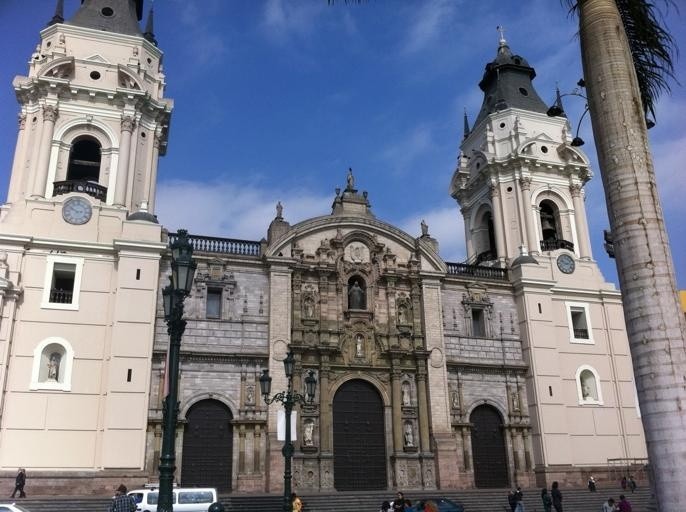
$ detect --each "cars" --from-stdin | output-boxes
[0,503,31,512]
[411,497,464,512]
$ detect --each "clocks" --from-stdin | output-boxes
[556,254,575,274]
[61,199,92,226]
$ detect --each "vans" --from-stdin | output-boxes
[126,488,218,512]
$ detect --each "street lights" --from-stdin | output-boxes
[259,352,317,512]
[547,78,655,146]
[157,229,198,512]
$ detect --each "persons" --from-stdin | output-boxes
[304,423,314,445]
[133,493,141,503]
[290,492,302,512]
[405,424,413,447]
[621,477,627,489]
[348,281,365,308]
[514,486,525,511]
[542,487,552,512]
[19,469,26,497]
[552,481,563,512]
[304,300,315,317]
[398,306,408,323]
[48,354,57,379]
[10,468,24,497]
[380,491,440,511]
[588,476,596,493]
[208,502,225,511]
[421,219,428,234]
[613,494,632,512]
[628,476,636,493]
[403,387,411,406]
[347,167,355,189]
[601,499,616,511]
[276,199,283,217]
[108,483,138,512]
[355,335,364,355]
[508,490,516,511]
